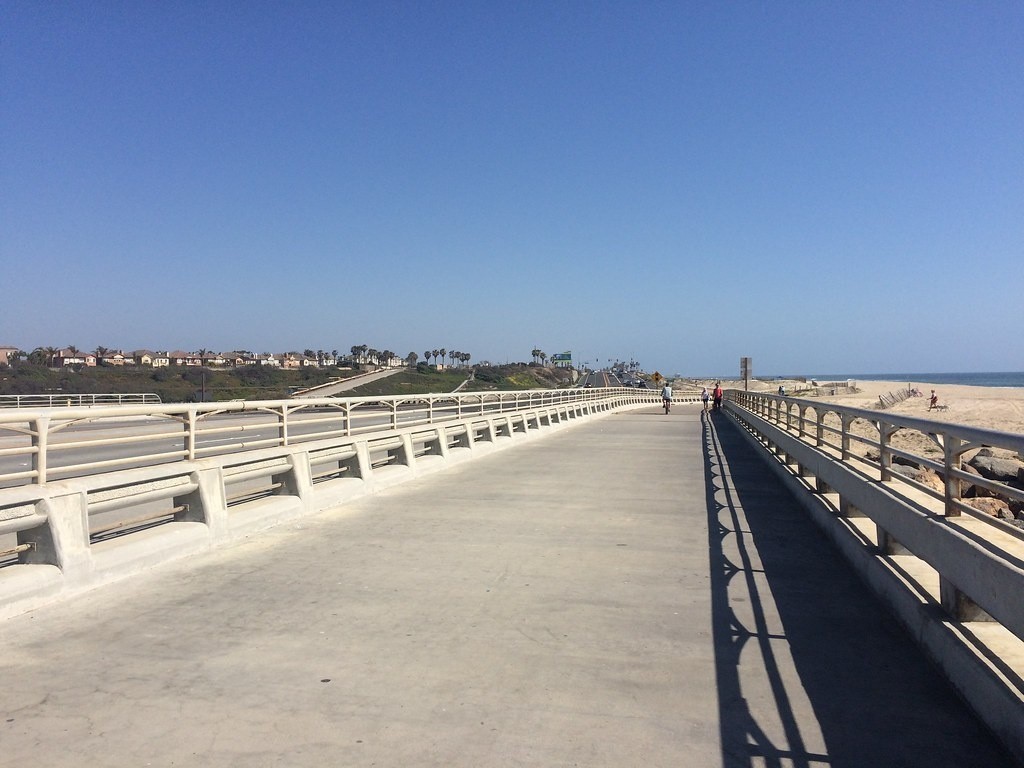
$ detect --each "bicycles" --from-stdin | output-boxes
[661,394,673,415]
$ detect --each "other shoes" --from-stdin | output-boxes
[663,405,665,408]
[668,408,670,412]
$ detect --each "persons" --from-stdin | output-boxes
[660,382,672,412]
[701,387,709,409]
[779,386,785,395]
[712,383,722,414]
[927,389,939,412]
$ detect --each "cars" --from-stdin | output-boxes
[590,369,646,389]
[585,382,593,388]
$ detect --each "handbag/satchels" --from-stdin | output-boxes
[933,396,938,403]
